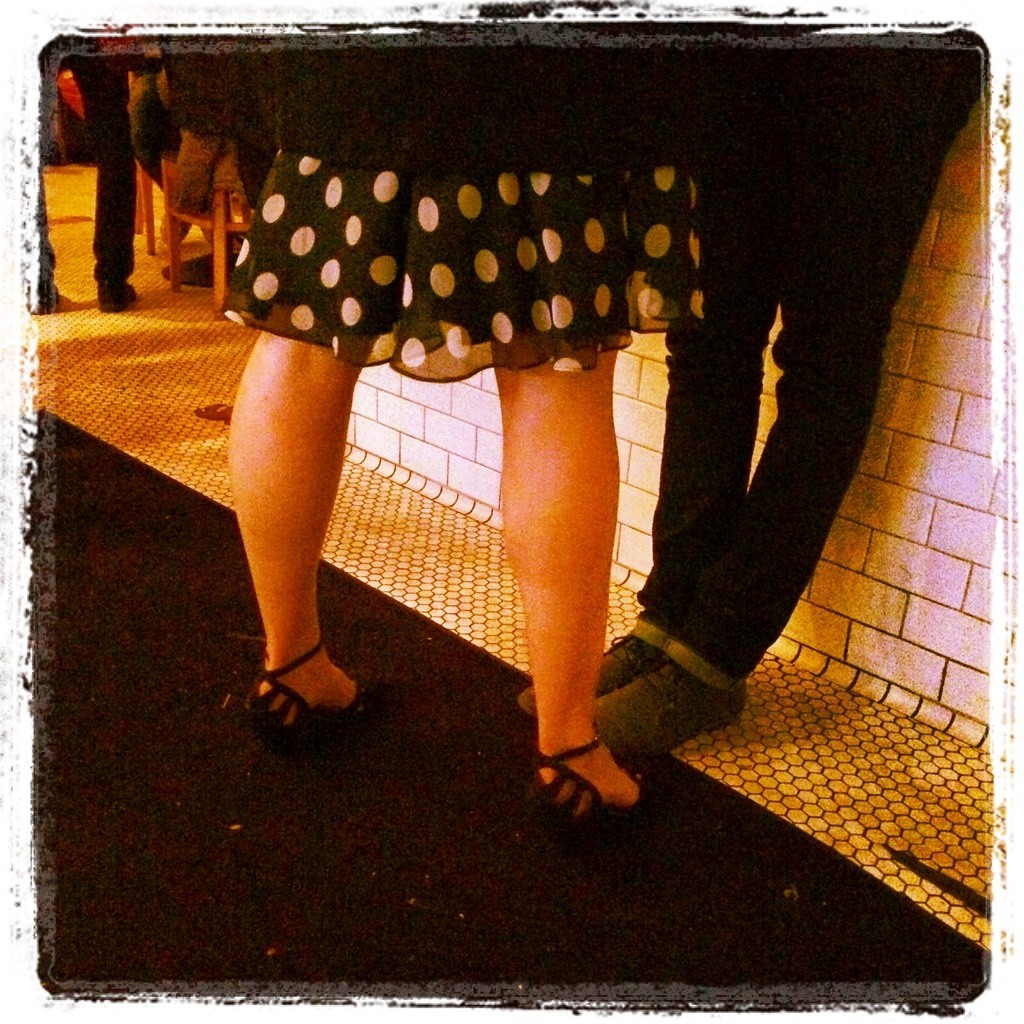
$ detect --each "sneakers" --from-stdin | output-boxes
[593,655,744,750]
[516,635,673,719]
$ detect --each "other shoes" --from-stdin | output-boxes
[97,279,136,313]
[39,280,58,313]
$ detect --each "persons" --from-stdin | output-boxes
[226,50,700,835]
[35,51,278,316]
[515,49,981,758]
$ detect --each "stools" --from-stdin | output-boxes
[162,147,266,315]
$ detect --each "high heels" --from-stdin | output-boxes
[534,737,674,859]
[250,638,385,751]
